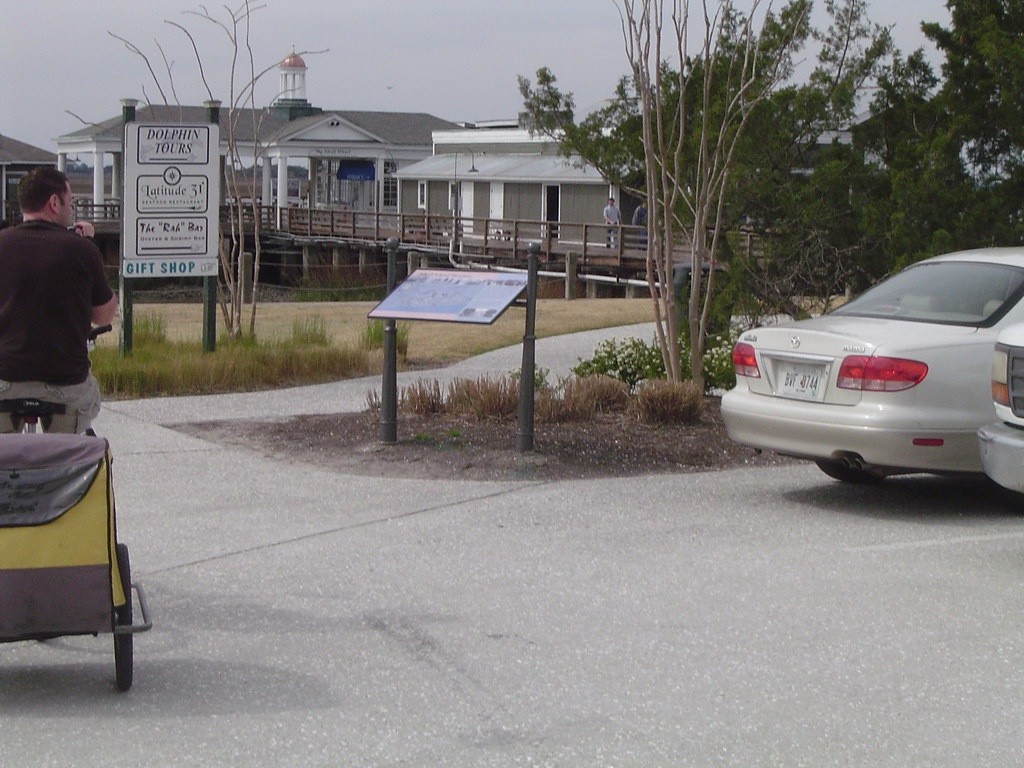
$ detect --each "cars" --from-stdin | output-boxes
[719,245,1024,486]
[979,323,1024,496]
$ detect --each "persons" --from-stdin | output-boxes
[604,198,623,248]
[632,199,648,252]
[0,167,116,434]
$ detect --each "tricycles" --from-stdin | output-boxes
[0,321,153,692]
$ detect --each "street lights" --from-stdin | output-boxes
[374,150,396,243]
[454,148,478,253]
